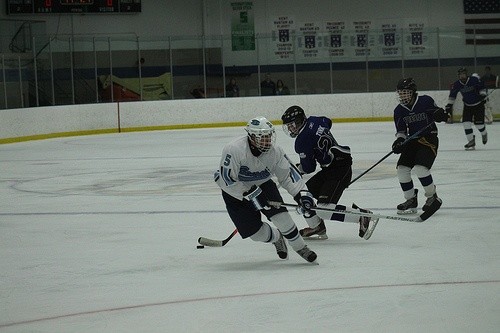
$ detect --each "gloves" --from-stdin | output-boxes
[242,184,271,210]
[434,109,450,122]
[299,190,313,217]
[392,138,405,154]
[444,104,453,115]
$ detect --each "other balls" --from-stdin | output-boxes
[197,246,204,249]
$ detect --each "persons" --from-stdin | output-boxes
[392,77,445,215]
[261,73,290,95]
[446,68,492,150]
[214,115,318,265]
[482,67,496,88]
[226,79,240,96]
[281,105,379,240]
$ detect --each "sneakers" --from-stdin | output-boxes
[351,203,378,240]
[463,135,475,150]
[296,247,319,266]
[396,189,419,215]
[422,193,437,211]
[482,134,487,145]
[299,219,330,240]
[274,229,288,260]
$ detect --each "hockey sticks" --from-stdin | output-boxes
[198,184,282,247]
[344,120,436,189]
[483,88,496,100]
[268,197,442,223]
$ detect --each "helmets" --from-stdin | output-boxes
[458,67,467,81]
[244,116,275,153]
[281,106,305,139]
[396,78,416,106]
